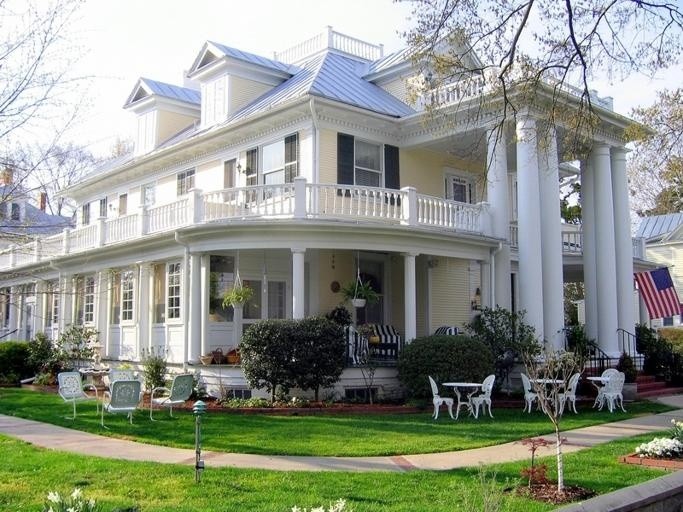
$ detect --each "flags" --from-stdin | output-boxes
[632,266,682,321]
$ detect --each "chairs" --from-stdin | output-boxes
[58,369,193,430]
[427,368,628,421]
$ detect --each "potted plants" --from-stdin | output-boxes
[222,286,254,310]
[140,345,168,406]
[341,277,379,307]
